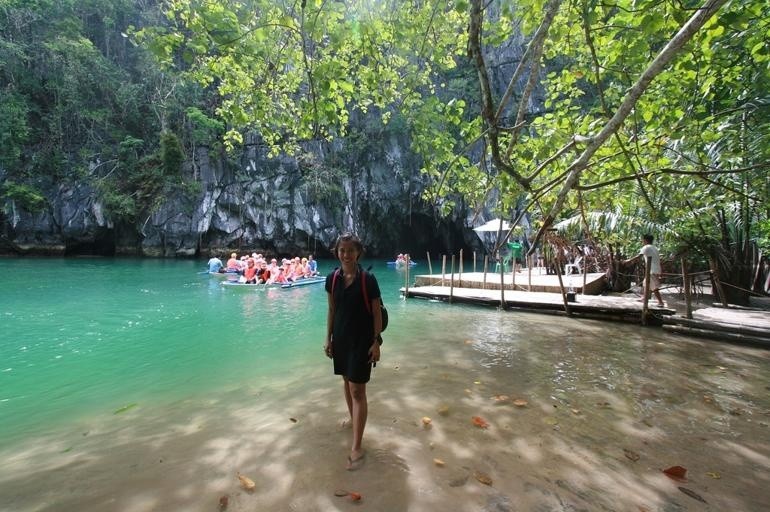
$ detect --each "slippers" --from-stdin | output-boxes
[348,455,364,471]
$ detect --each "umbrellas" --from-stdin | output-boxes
[470,216,522,259]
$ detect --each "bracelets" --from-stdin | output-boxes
[373,336,379,341]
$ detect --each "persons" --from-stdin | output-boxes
[621,233,665,308]
[396,264,411,274]
[535,246,548,272]
[395,251,405,263]
[403,253,415,263]
[245,289,310,318]
[323,230,383,471]
[208,251,320,286]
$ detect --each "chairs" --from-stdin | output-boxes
[495,255,510,273]
[565,255,582,277]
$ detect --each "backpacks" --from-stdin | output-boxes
[331,269,387,332]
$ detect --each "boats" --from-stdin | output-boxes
[220,276,328,288]
[386,260,417,266]
[209,266,237,275]
